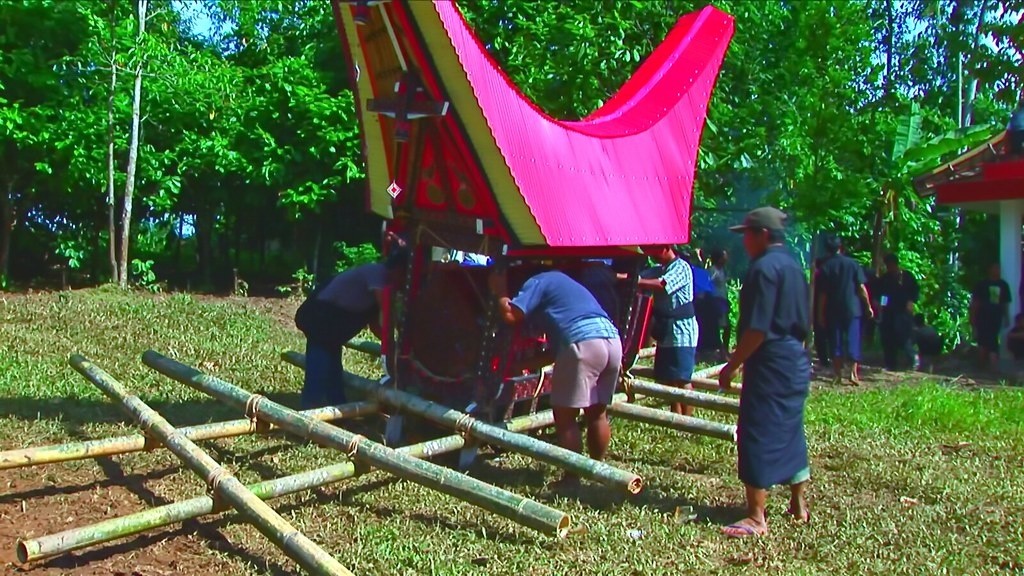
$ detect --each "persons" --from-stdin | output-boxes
[810,233,944,387]
[969,261,1024,375]
[719,207,810,538]
[488,265,624,493]
[637,245,732,418]
[295,247,408,429]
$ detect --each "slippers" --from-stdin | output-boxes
[781,508,810,524]
[724,515,768,537]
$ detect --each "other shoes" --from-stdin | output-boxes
[850,373,859,387]
[547,477,581,488]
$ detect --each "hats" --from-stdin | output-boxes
[827,237,842,247]
[731,207,786,230]
